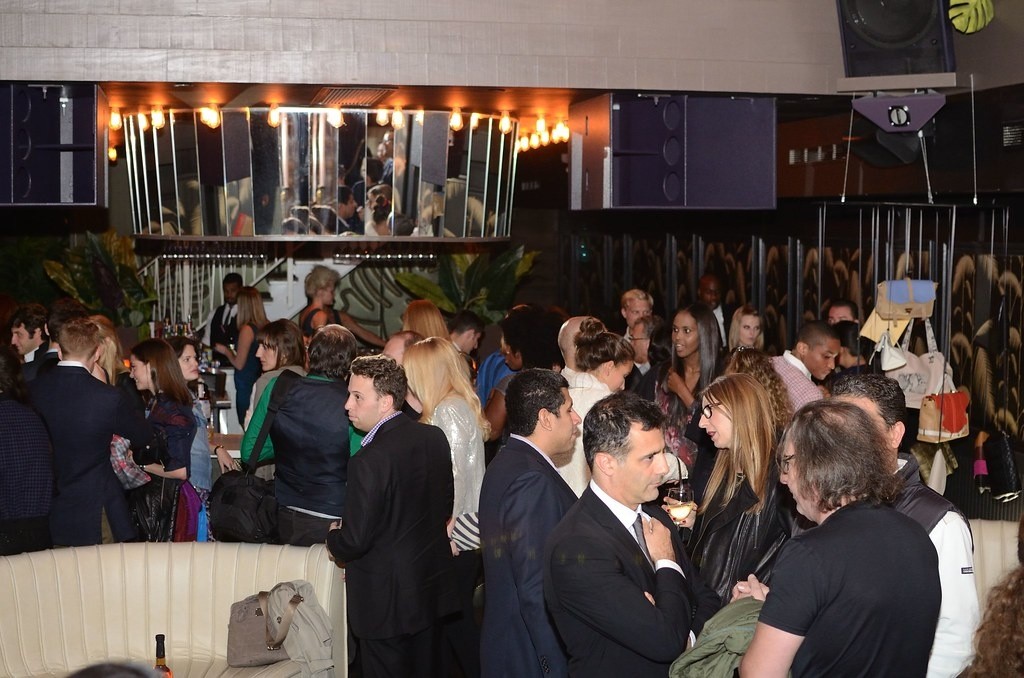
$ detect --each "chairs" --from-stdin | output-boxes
[910,441,980,514]
[969,517,1020,618]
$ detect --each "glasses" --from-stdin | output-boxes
[628,334,647,342]
[702,401,724,418]
[781,448,799,474]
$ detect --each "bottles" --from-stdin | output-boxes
[164,315,196,338]
[153,634,173,677]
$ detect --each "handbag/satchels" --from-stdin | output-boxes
[226,591,296,667]
[876,280,937,320]
[884,318,954,409]
[209,470,278,543]
[875,334,907,372]
[917,373,969,444]
[910,440,959,485]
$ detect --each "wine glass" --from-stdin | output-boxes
[668,488,694,530]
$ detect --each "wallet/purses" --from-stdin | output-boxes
[983,433,1022,502]
[974,431,990,494]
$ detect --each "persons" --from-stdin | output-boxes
[0,114,1024,678]
[328,356,458,678]
[478,368,582,677]
[544,391,722,677]
[728,399,943,678]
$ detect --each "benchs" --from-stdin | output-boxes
[0,540,350,678]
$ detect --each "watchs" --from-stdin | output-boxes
[214,444,225,455]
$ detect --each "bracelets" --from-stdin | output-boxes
[139,464,145,471]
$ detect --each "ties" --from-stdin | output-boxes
[632,513,652,567]
[224,305,233,325]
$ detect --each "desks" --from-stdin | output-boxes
[207,434,245,488]
[144,391,231,434]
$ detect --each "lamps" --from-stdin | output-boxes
[108,106,572,163]
